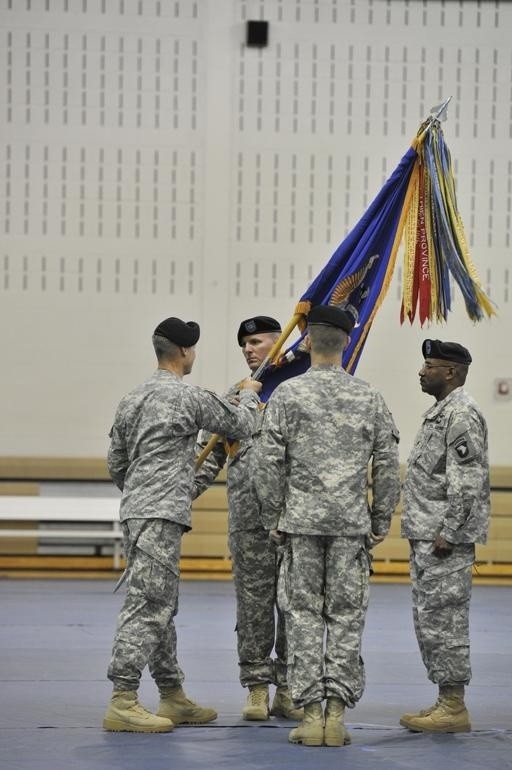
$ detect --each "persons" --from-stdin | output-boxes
[400,338,492,736]
[256,307,399,749]
[188,311,311,726]
[97,315,263,737]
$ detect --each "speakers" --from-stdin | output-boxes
[247,20,269,45]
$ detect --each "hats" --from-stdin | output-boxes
[307,305,352,335]
[154,317,200,347]
[238,316,282,347]
[422,339,472,365]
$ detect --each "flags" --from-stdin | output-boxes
[294,140,422,377]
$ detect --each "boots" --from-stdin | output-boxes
[243,683,271,721]
[288,702,325,746]
[399,686,445,728]
[155,685,218,725]
[407,684,471,734]
[102,689,174,733]
[270,686,305,721]
[323,697,351,747]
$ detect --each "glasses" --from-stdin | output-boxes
[422,364,452,369]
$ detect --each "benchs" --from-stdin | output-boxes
[1,492,128,572]
